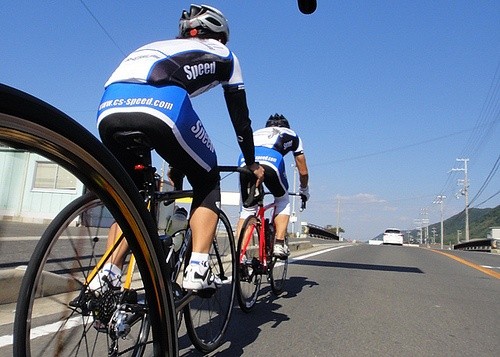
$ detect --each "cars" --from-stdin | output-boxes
[382,228,404,246]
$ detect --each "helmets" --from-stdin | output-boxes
[266,113,290,129]
[180,4,229,43]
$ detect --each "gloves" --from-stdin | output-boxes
[298,187,309,201]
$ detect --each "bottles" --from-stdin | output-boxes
[264,218,274,252]
[159,234,176,273]
[165,207,188,252]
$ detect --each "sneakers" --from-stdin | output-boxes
[273,245,290,256]
[183,261,223,291]
[239,255,249,276]
[87,270,122,294]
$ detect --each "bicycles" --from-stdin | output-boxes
[0,81,311,357]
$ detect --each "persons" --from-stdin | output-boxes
[237,113,309,278]
[84,4,265,329]
